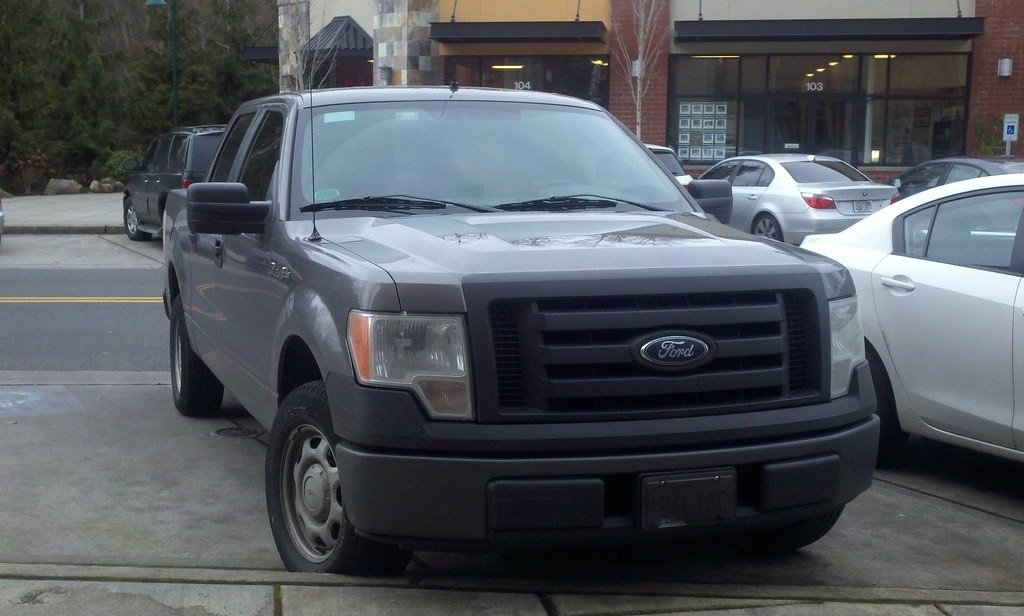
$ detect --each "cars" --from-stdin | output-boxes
[889,157,1024,236]
[801,175,1024,466]
[696,152,899,247]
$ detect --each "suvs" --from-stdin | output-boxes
[643,144,694,189]
[162,87,880,575]
[122,124,226,241]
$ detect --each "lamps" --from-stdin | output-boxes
[632,59,646,78]
[378,64,392,79]
[280,72,292,88]
[997,57,1013,77]
